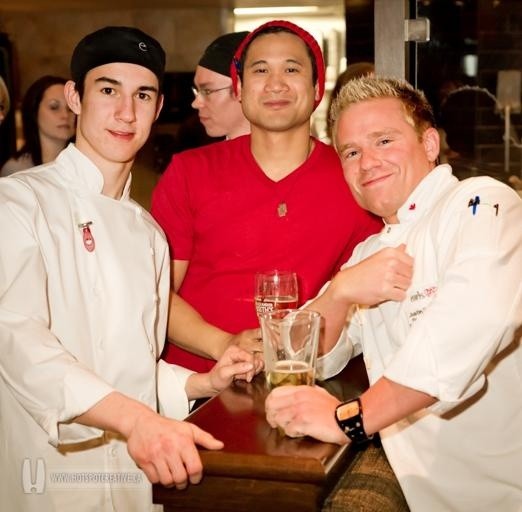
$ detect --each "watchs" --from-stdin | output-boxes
[333,397,376,445]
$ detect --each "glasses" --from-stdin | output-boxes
[192,84,233,100]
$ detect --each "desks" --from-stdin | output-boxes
[153,375,370,511]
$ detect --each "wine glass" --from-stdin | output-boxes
[253,269,299,360]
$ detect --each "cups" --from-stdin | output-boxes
[254,309,323,439]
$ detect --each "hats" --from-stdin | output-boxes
[198,31,251,78]
[71,26,165,90]
[231,20,325,113]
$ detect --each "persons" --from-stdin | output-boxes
[0,73,77,178]
[191,30,254,141]
[149,19,385,408]
[326,61,374,139]
[265,73,522,511]
[440,84,522,197]
[1,23,270,512]
